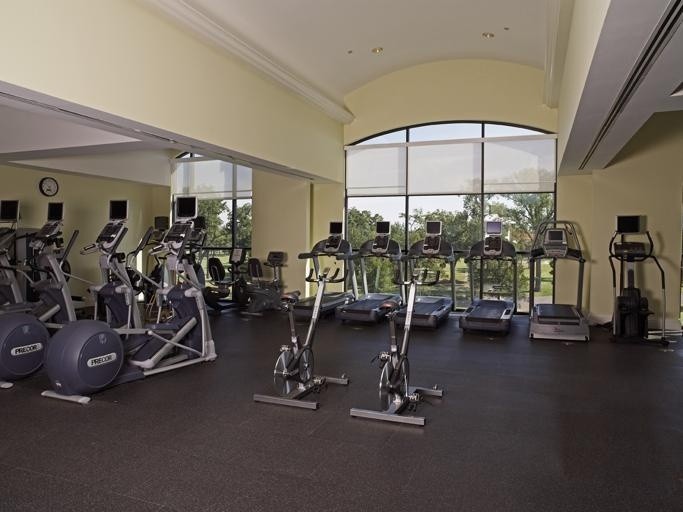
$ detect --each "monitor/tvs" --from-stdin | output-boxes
[155,216,169,229]
[485,220,502,235]
[615,214,643,235]
[228,247,246,265]
[109,199,129,221]
[543,229,568,245]
[375,221,391,236]
[47,201,65,221]
[328,220,344,235]
[190,215,206,229]
[0,200,20,222]
[425,220,442,236]
[174,194,198,219]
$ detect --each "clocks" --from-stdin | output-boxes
[39,177,59,196]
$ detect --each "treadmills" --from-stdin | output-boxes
[289,222,352,320]
[389,221,455,328]
[529,221,590,344]
[459,221,517,336]
[336,221,402,327]
